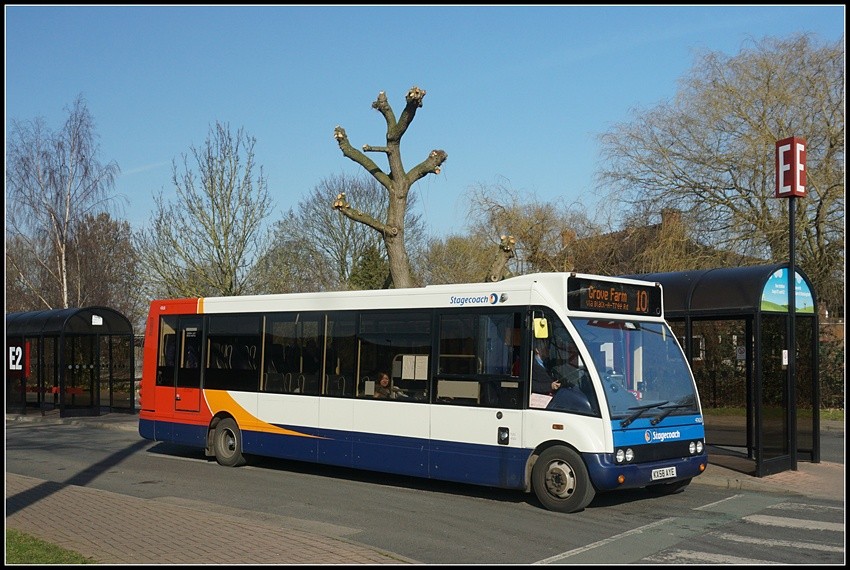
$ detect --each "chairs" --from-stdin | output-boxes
[164,334,372,398]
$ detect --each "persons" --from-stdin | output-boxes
[531,341,564,396]
[372,372,395,397]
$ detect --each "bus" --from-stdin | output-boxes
[138,271,709,512]
